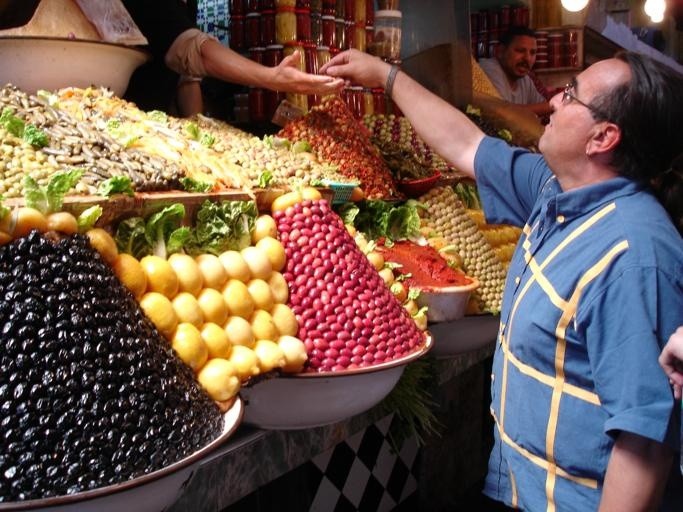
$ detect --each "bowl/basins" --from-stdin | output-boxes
[241,331,436,430]
[1,35,149,100]
[426,312,501,360]
[393,167,443,198]
[415,276,480,323]
[1,391,245,511]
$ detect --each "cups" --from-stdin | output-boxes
[547,33,563,66]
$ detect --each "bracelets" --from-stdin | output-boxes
[385,57,404,99]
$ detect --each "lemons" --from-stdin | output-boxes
[466,209,523,273]
[0,187,322,413]
[344,225,428,332]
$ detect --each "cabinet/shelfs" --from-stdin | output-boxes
[476,20,583,73]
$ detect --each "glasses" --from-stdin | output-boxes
[562,83,612,121]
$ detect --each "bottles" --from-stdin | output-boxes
[469,5,511,59]
[227,1,402,139]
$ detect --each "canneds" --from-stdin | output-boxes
[228,0,404,123]
[471,4,528,59]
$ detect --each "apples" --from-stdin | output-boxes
[498,129,512,142]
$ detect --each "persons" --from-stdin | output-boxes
[316,48,683,511]
[122,1,346,130]
[476,26,554,124]
[658,323,683,400]
